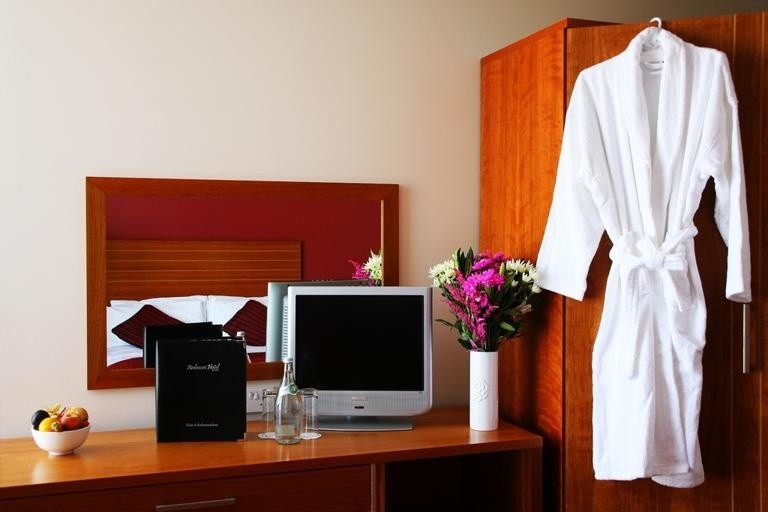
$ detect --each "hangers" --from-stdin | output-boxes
[634,16,675,67]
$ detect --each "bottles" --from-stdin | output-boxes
[275,358,301,444]
[237,330,251,363]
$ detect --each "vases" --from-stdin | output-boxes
[467,347,501,433]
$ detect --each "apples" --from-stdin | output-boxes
[62,413,79,430]
[72,407,88,427]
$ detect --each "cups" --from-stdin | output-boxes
[263,389,276,437]
[301,389,319,439]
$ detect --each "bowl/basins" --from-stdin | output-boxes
[31,421,92,456]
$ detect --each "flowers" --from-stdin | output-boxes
[347,248,383,287]
[427,247,542,354]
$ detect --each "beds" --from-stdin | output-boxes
[96,237,302,388]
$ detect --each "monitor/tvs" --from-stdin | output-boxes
[265,279,382,364]
[287,286,434,431]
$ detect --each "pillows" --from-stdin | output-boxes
[110,297,203,327]
[111,304,184,349]
[206,295,268,331]
[222,299,267,347]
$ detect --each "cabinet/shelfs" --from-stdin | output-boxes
[0,404,544,511]
[481,12,767,511]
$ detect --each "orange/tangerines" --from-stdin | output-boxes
[38,418,61,432]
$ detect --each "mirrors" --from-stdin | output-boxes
[84,176,399,391]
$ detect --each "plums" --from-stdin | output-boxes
[31,410,49,430]
